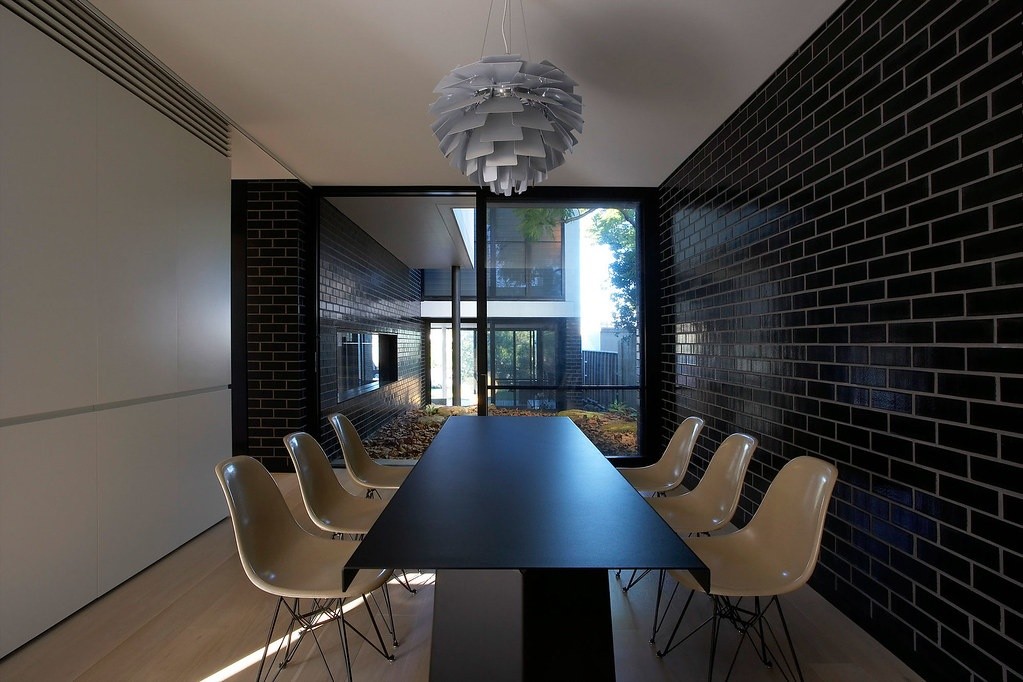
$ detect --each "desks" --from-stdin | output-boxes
[341,415,711,682]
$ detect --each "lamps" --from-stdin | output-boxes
[428,1,585,197]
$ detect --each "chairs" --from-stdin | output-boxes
[215,412,414,682]
[615,417,838,682]
